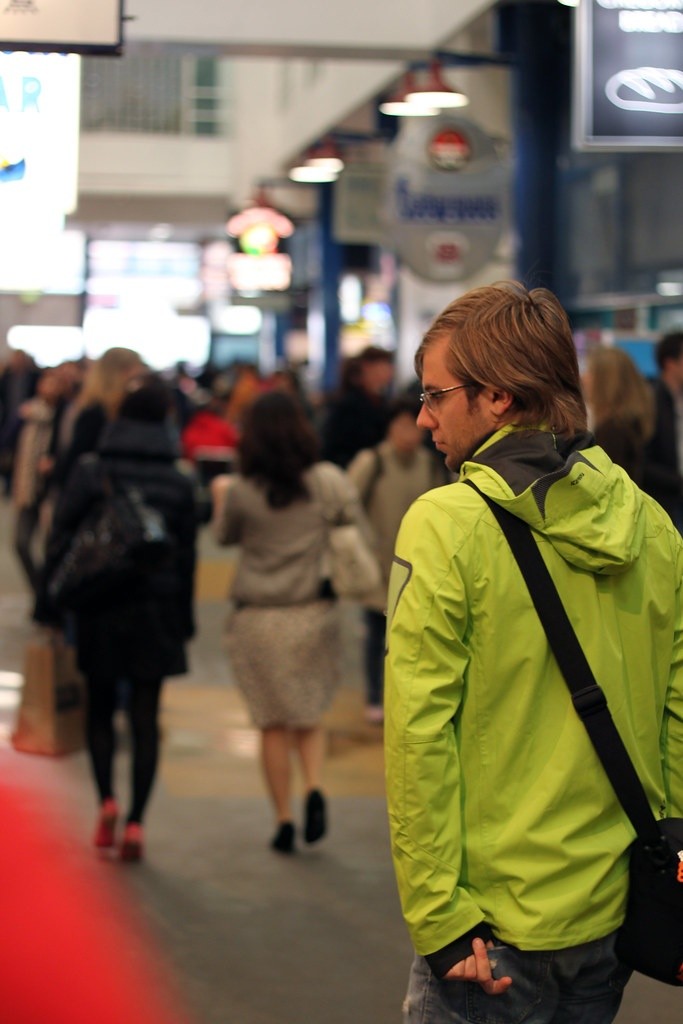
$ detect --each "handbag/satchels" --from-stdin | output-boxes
[615,817,683,986]
[13,626,81,756]
[320,464,378,600]
[43,473,151,605]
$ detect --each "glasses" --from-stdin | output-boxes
[420,384,482,414]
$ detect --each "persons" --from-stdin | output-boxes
[346,398,434,723]
[212,389,376,853]
[576,327,683,536]
[176,346,399,523]
[383,280,683,1024]
[0,347,148,502]
[30,386,200,863]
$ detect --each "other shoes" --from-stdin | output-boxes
[304,791,325,845]
[272,822,293,851]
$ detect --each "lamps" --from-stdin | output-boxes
[290,56,471,183]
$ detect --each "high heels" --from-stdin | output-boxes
[121,823,144,862]
[95,799,118,848]
